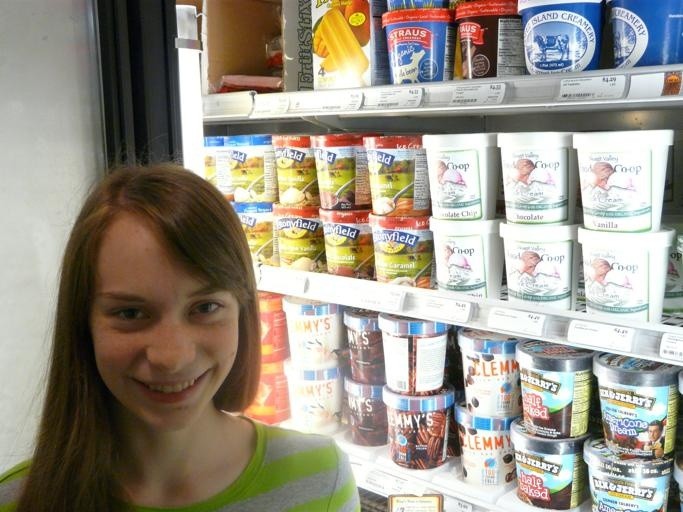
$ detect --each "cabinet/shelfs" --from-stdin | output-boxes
[198,0,683,512]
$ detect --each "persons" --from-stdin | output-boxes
[0,160,361,512]
[641,420,665,459]
